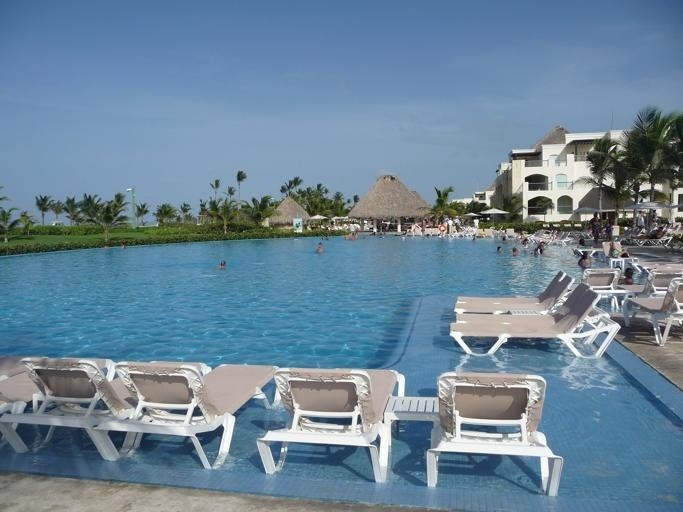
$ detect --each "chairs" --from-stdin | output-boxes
[448,223,682,358]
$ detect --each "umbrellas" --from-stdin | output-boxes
[306,214,328,225]
[622,201,681,225]
[479,207,511,226]
[575,205,597,221]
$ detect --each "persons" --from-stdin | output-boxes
[424,209,682,284]
[218,260,226,269]
[302,220,398,240]
[119,239,126,249]
[315,242,324,253]
